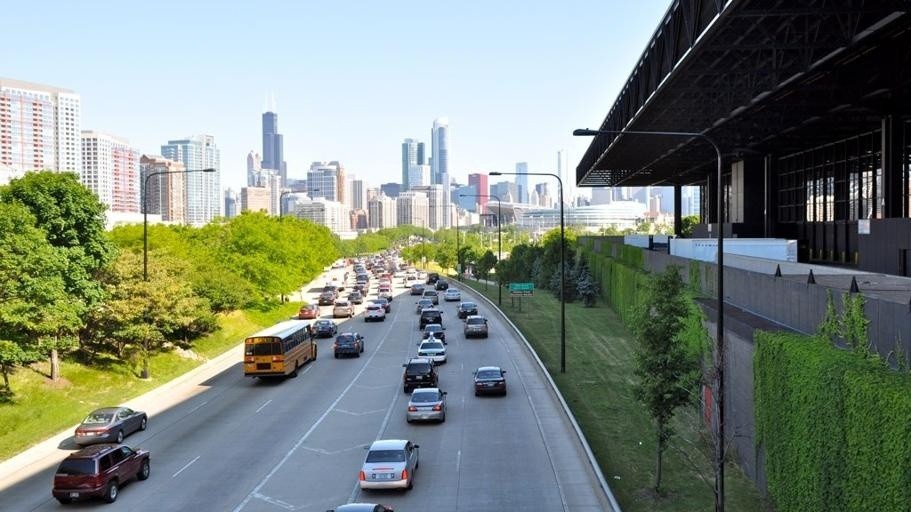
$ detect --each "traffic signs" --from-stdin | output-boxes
[509,282,535,297]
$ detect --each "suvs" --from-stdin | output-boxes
[333,331,365,360]
[418,307,445,329]
[402,356,443,392]
[49,442,153,505]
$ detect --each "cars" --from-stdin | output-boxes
[416,331,450,364]
[326,500,396,511]
[471,365,509,398]
[462,314,490,339]
[312,318,339,338]
[454,300,481,320]
[72,405,150,446]
[318,241,462,323]
[357,437,421,491]
[299,304,321,319]
[419,322,449,343]
[405,386,449,424]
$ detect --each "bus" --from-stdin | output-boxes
[240,317,319,381]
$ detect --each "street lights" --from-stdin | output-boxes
[488,171,568,374]
[297,207,327,219]
[460,193,504,306]
[279,187,322,302]
[140,166,220,381]
[571,126,732,511]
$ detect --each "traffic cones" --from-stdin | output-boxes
[774,263,783,276]
[849,275,861,293]
[808,268,818,283]
